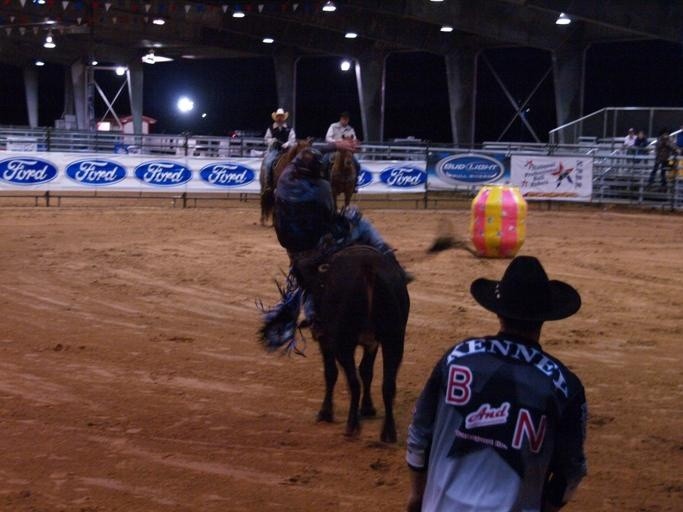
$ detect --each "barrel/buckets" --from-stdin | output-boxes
[466,184,530,257]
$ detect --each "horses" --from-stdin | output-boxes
[328,134,357,212]
[259,137,315,227]
[302,240,412,444]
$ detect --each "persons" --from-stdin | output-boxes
[623,128,637,147]
[647,129,682,194]
[325,203,384,253]
[275,139,356,253]
[326,111,358,144]
[627,130,649,162]
[263,108,296,183]
[409,255,587,512]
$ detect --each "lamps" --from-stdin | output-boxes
[322,0,336,11]
[43,29,57,49]
[555,12,571,24]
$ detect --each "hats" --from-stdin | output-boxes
[272,108,289,121]
[470,256,581,320]
